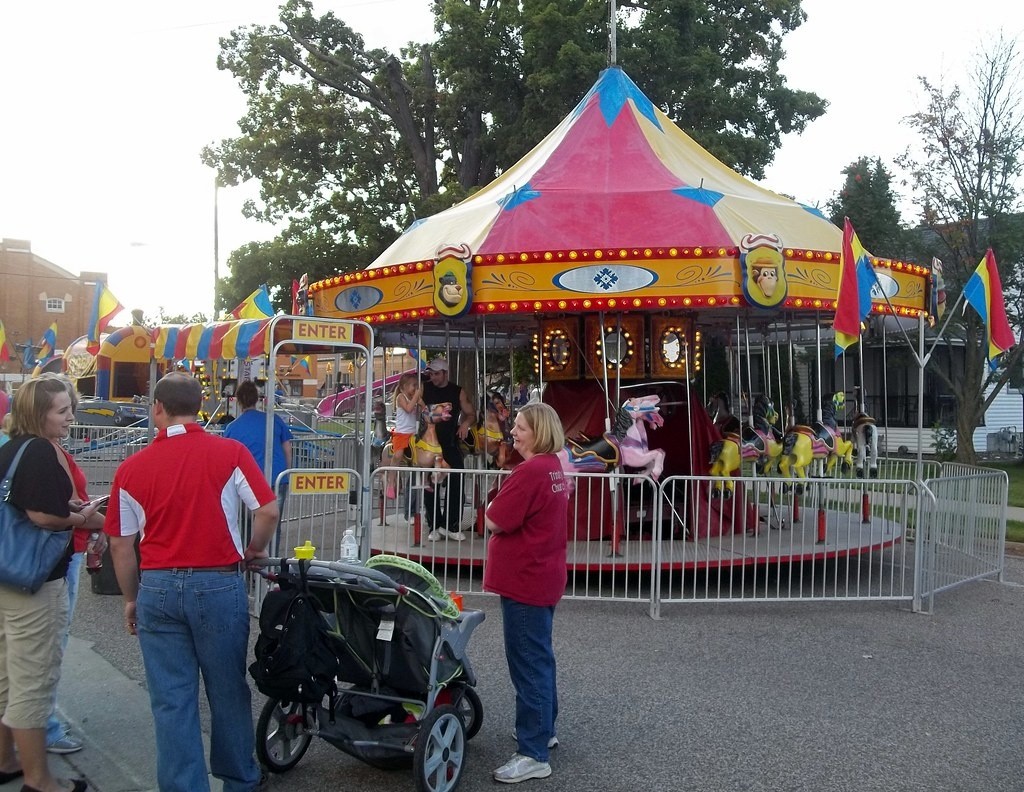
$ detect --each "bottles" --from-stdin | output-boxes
[87,533,102,575]
[340,529,358,564]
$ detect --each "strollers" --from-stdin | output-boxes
[246,542,486,792]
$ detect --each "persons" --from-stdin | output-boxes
[223,379,294,590]
[102,372,281,792]
[482,403,569,783]
[0,370,107,792]
[383,357,476,542]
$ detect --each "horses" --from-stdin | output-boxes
[455,388,513,468]
[373,402,451,497]
[554,394,666,495]
[706,389,880,501]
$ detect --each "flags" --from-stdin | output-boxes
[36,319,58,367]
[962,246,1015,372]
[232,283,275,322]
[290,354,298,365]
[22,337,35,370]
[85,276,125,356]
[0,319,10,363]
[830,215,877,362]
[409,348,427,370]
[300,354,313,376]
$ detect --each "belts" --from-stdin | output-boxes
[168,560,238,572]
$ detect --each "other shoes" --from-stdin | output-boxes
[20,778,87,792]
[386,486,395,499]
[47,735,83,753]
[251,769,275,792]
[55,720,72,733]
[0,770,23,785]
[425,486,434,493]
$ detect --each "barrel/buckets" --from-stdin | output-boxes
[90,500,142,595]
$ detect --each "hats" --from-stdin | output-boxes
[426,358,449,372]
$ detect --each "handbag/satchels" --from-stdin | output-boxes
[0,437,73,595]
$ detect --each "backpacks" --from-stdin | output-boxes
[249,558,341,727]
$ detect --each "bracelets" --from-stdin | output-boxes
[80,513,87,528]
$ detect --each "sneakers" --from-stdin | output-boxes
[428,529,441,541]
[439,526,466,541]
[493,752,551,784]
[512,728,559,749]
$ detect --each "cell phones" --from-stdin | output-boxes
[82,494,110,507]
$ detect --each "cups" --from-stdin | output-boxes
[293,541,316,559]
[450,592,463,611]
